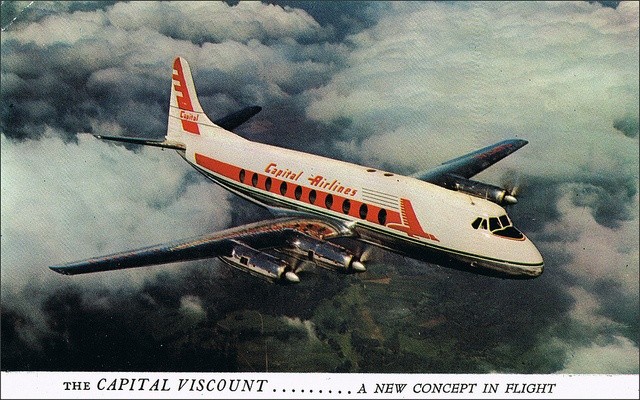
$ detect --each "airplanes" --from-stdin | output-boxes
[48,55,545,285]
